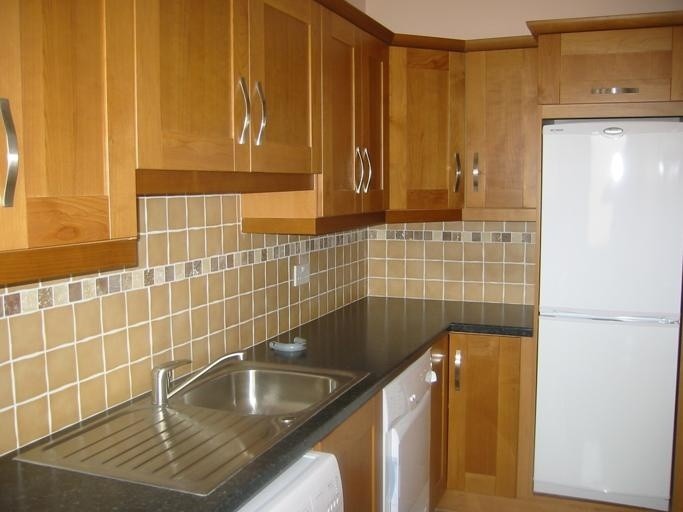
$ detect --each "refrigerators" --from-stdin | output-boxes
[532,117,683,512]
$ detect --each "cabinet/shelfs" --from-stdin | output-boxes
[318,390,382,511]
[525,9,683,120]
[431,327,530,511]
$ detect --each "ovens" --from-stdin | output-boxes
[381,347,432,512]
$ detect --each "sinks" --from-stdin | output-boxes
[168,360,371,422]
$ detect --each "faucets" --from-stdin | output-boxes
[151,350,248,406]
